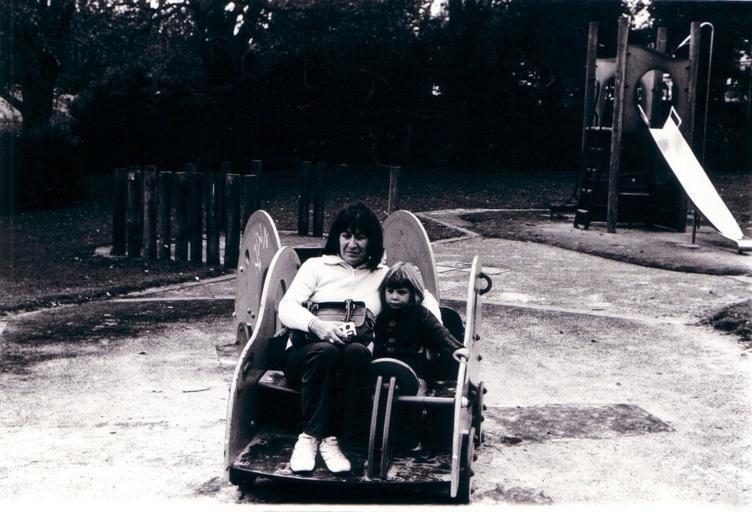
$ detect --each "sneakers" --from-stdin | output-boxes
[319,436,352,474]
[289,431,318,473]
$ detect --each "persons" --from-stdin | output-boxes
[373,261,470,462]
[278,203,443,474]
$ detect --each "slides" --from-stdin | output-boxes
[638,104,752,252]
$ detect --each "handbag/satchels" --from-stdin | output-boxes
[288,299,376,348]
